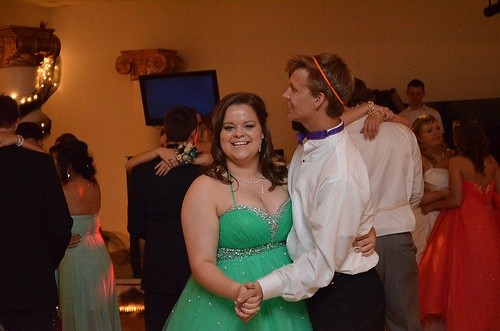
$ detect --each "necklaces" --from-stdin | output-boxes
[229,167,265,194]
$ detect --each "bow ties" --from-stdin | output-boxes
[291,119,345,145]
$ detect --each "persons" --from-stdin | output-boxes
[0,94,74,331]
[397,79,444,135]
[234,53,387,331]
[125,107,225,331]
[50,132,125,331]
[163,94,376,331]
[340,78,422,331]
[411,115,456,265]
[17,122,44,150]
[421,113,500,331]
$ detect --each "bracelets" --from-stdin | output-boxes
[18,136,24,147]
[17,135,20,145]
[369,110,383,120]
[367,101,373,113]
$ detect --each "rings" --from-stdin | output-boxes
[170,160,174,163]
[391,111,393,113]
[165,164,168,168]
[70,242,72,244]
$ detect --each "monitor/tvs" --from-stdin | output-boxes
[139,69,220,126]
